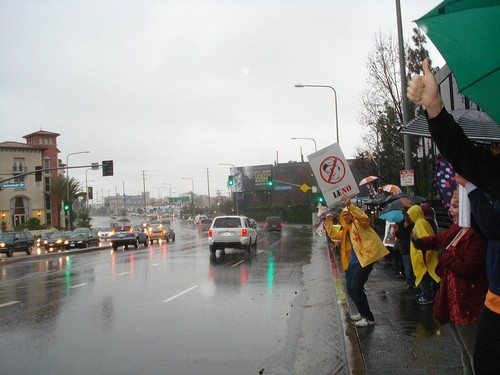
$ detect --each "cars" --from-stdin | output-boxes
[149,225,176,243]
[208,215,258,254]
[141,213,171,228]
[97,227,112,241]
[186,214,213,233]
[70,228,100,248]
[250,219,258,232]
[37,231,72,250]
[264,216,284,233]
[110,226,149,249]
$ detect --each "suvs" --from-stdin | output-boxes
[0,231,34,257]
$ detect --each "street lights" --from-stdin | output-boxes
[182,177,195,217]
[295,84,339,144]
[219,164,238,216]
[67,151,90,229]
[291,138,317,152]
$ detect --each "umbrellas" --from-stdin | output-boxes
[412,0,500,126]
[381,197,427,213]
[378,210,404,221]
[358,175,378,185]
[363,199,378,206]
[372,192,387,204]
[400,109,500,142]
[378,184,402,194]
[383,193,407,201]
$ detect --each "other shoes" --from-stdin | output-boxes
[355,318,374,326]
[418,295,435,305]
[350,313,363,320]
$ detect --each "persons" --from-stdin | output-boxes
[323,57,500,375]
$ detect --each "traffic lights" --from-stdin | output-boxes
[319,197,323,202]
[64,201,69,211]
[229,175,233,184]
[268,176,272,185]
